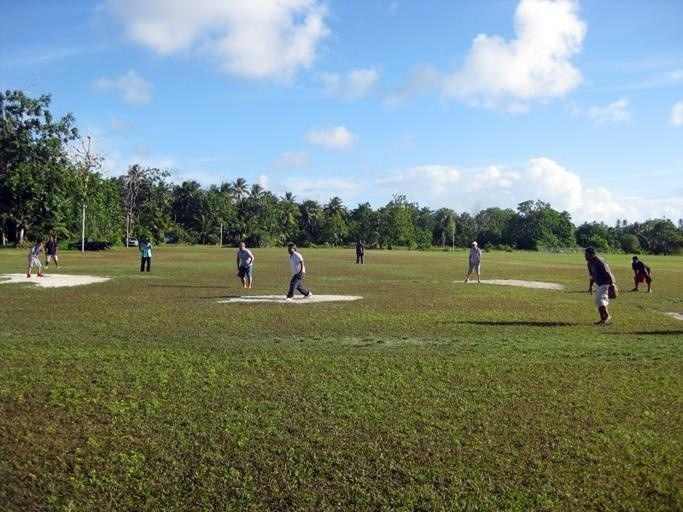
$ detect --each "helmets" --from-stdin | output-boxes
[471,241,478,248]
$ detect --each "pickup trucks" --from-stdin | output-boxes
[68,238,112,251]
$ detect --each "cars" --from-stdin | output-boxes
[125,237,138,247]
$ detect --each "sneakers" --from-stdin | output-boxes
[304,292,312,299]
[285,297,294,301]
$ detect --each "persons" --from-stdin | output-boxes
[464,241,481,284]
[632,256,652,293]
[27,238,48,278]
[585,247,616,327]
[236,242,255,288]
[43,233,60,269]
[139,237,152,272]
[355,239,365,264]
[284,244,312,301]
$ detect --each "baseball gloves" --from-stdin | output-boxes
[607,285,619,298]
[237,265,245,276]
